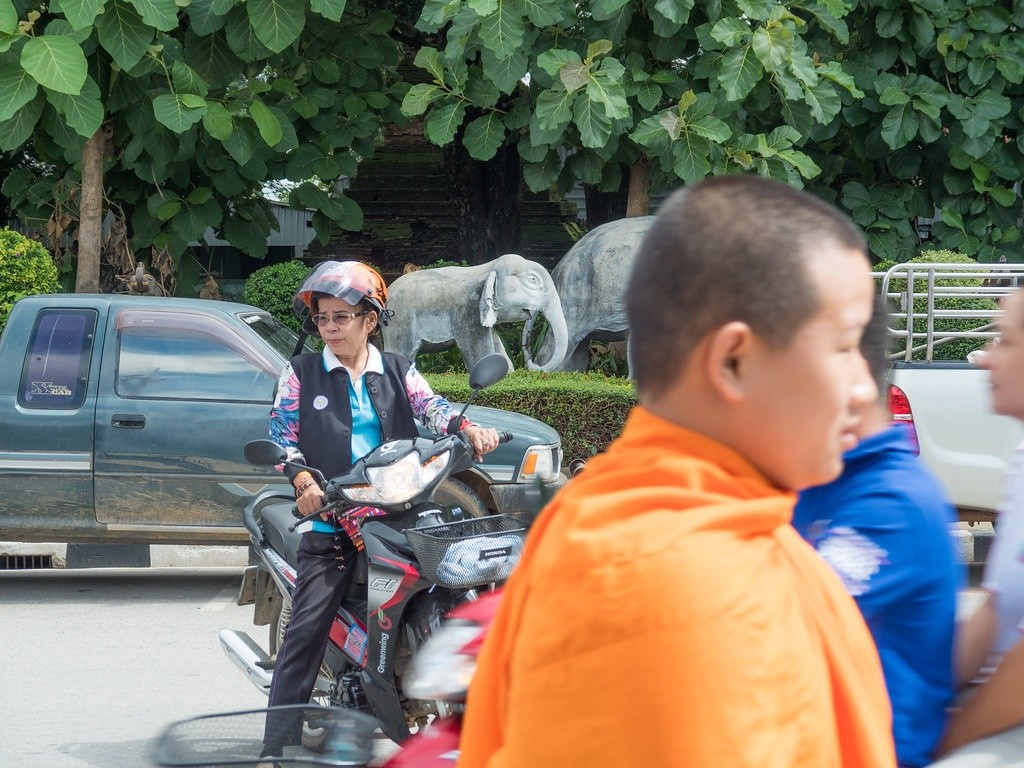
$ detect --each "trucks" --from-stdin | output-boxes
[867,260,1023,530]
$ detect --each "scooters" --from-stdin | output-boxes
[378,584,507,768]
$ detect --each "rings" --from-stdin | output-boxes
[484,442,489,447]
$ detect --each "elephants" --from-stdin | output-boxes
[380,214,659,379]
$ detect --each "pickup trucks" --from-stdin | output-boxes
[0,294,576,543]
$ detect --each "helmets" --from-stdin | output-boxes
[306,260,389,318]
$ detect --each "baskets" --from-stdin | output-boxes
[403,510,526,587]
[152,704,381,768]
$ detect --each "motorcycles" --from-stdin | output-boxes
[216,438,530,749]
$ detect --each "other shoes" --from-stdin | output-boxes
[255,756,282,768]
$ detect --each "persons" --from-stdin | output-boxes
[255,258,499,767]
[455,172,899,767]
[792,287,957,768]
[928,286,1024,768]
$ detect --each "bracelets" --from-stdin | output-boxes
[295,476,316,499]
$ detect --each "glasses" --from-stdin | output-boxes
[311,311,369,327]
